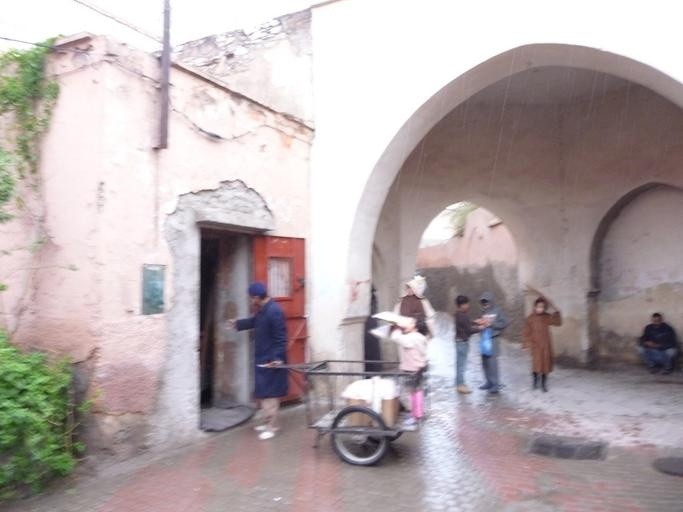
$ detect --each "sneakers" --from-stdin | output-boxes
[453,381,545,393]
[253,422,280,440]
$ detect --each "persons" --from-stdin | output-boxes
[224,281,287,442]
[452,293,485,396]
[391,320,429,432]
[522,295,563,392]
[478,293,507,396]
[364,283,384,377]
[637,312,680,375]
[400,272,436,336]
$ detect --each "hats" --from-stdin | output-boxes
[397,315,417,332]
[405,276,428,300]
[246,282,266,297]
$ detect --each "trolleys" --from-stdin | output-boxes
[252,359,431,466]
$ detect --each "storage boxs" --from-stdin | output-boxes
[347,397,400,427]
